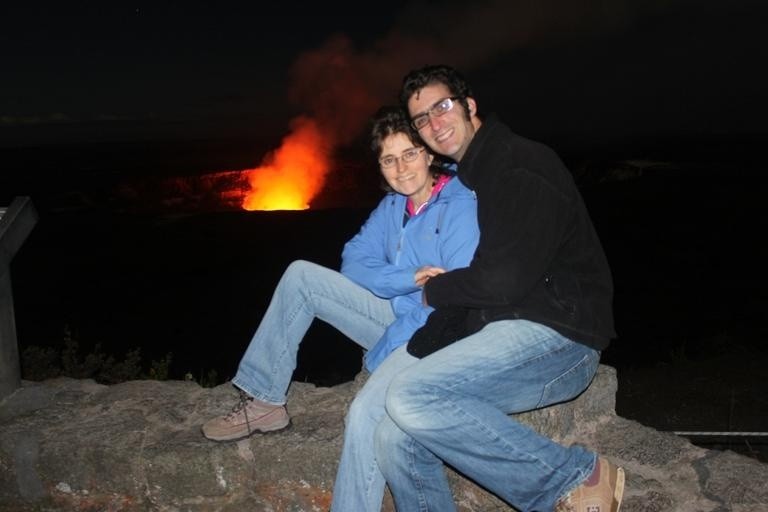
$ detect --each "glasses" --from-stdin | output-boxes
[376,148,426,168]
[411,96,457,132]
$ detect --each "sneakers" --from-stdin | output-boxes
[555,455,626,512]
[202,397,290,441]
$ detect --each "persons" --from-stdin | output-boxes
[200,102,479,511]
[375,65,626,511]
